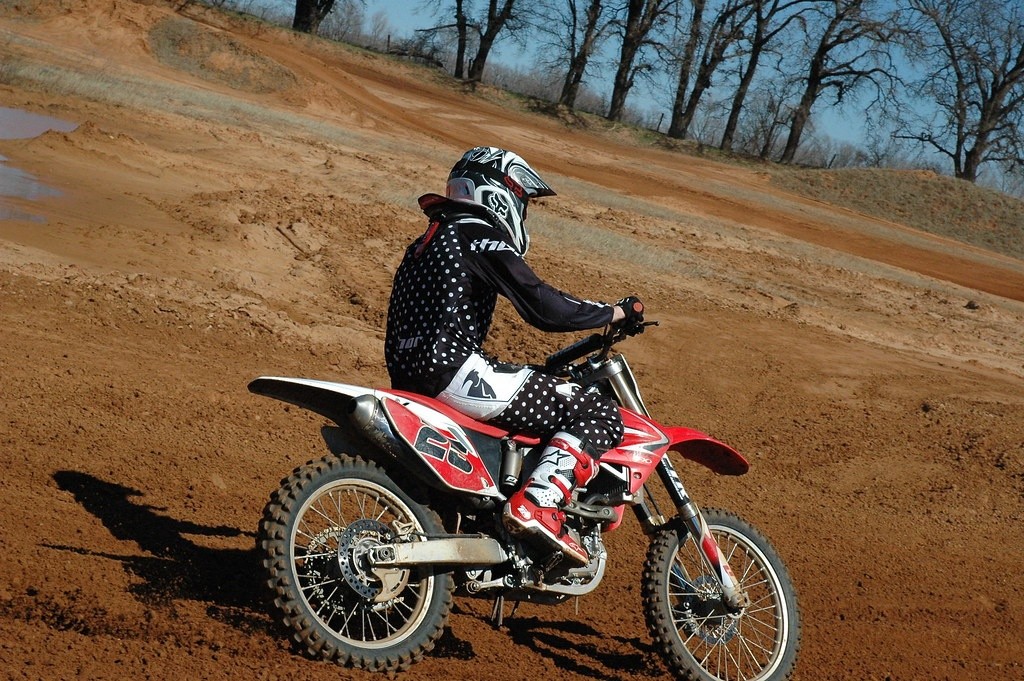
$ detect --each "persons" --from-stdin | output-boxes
[383,146,646,569]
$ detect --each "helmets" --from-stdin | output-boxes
[446,146,557,258]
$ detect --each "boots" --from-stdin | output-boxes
[501,432,600,566]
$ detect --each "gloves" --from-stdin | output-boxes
[608,296,644,337]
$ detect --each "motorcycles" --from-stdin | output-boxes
[246,300,803,681]
